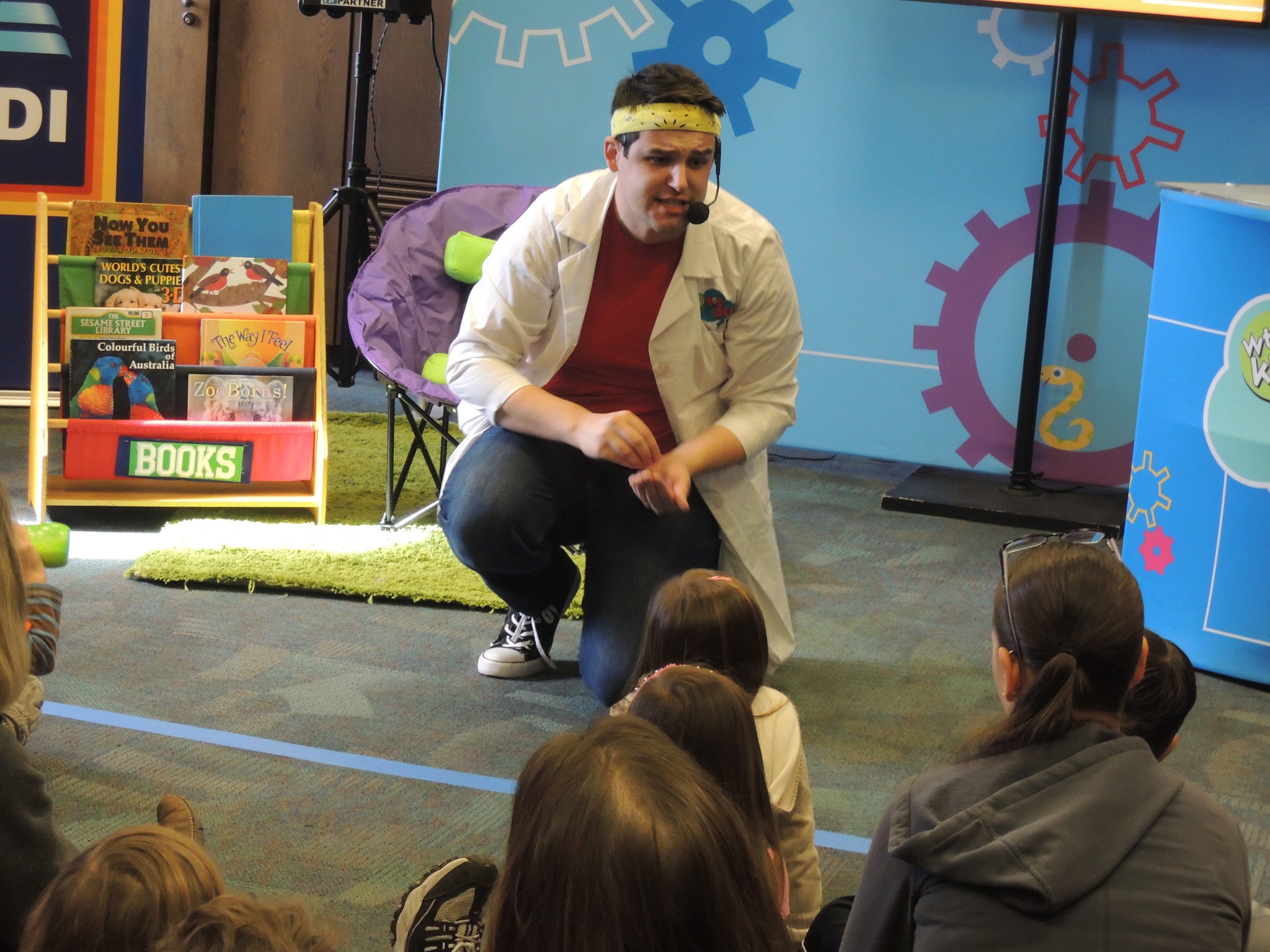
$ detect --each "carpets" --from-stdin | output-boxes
[123,411,586,620]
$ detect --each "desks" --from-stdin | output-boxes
[1121,182,1270,689]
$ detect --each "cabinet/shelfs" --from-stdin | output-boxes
[27,192,327,524]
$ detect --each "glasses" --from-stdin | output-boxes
[997,525,1122,685]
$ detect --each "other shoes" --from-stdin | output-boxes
[156,794,205,853]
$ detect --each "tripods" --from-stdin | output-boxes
[315,11,388,387]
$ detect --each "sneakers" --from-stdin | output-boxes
[392,851,502,952]
[477,552,580,681]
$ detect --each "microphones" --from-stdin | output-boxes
[687,147,721,224]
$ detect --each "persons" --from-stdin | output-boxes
[1,486,1252,951]
[436,64,803,710]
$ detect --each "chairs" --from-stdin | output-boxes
[346,184,548,530]
